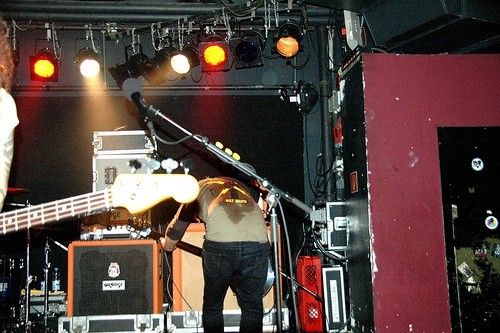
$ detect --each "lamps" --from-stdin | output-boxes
[271,0,304,65]
[107,7,265,91]
[29,29,61,82]
[74,30,100,78]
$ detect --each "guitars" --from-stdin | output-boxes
[0,156,201,235]
[126,216,275,298]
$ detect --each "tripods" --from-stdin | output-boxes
[4,229,56,333]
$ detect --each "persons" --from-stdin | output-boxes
[0,23,23,213]
[159,176,271,333]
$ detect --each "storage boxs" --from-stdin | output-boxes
[92,155,151,225]
[322,267,347,333]
[92,131,157,155]
[313,202,348,250]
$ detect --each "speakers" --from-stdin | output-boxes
[67,239,163,317]
[172,221,281,312]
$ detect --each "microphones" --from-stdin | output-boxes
[123,78,156,137]
